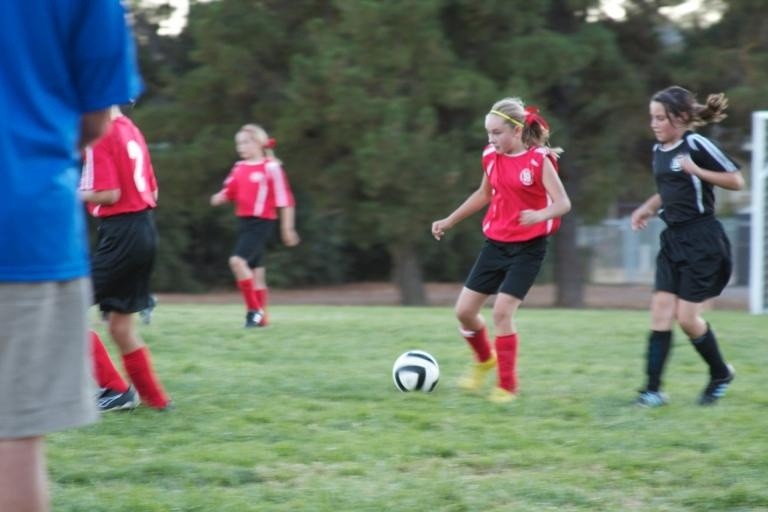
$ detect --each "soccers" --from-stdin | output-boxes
[392,349,440,391]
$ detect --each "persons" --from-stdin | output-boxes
[430,95,573,407]
[625,81,747,410]
[209,122,303,330]
[72,104,181,416]
[1,0,148,511]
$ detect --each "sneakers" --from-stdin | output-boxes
[140,295,157,326]
[243,309,264,328]
[459,351,498,390]
[95,385,142,413]
[700,363,736,405]
[488,386,518,404]
[632,392,668,409]
